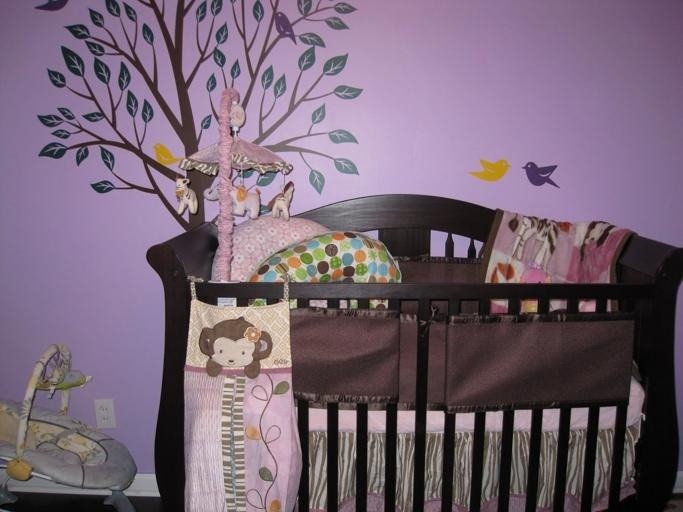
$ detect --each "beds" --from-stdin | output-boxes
[146,194,683,511]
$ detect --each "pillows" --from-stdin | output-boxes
[211,215,330,283]
[247,232,402,308]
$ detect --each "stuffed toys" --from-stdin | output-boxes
[173,177,199,215]
[204,181,261,219]
[267,181,296,221]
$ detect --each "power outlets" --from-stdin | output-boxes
[95,399,116,429]
